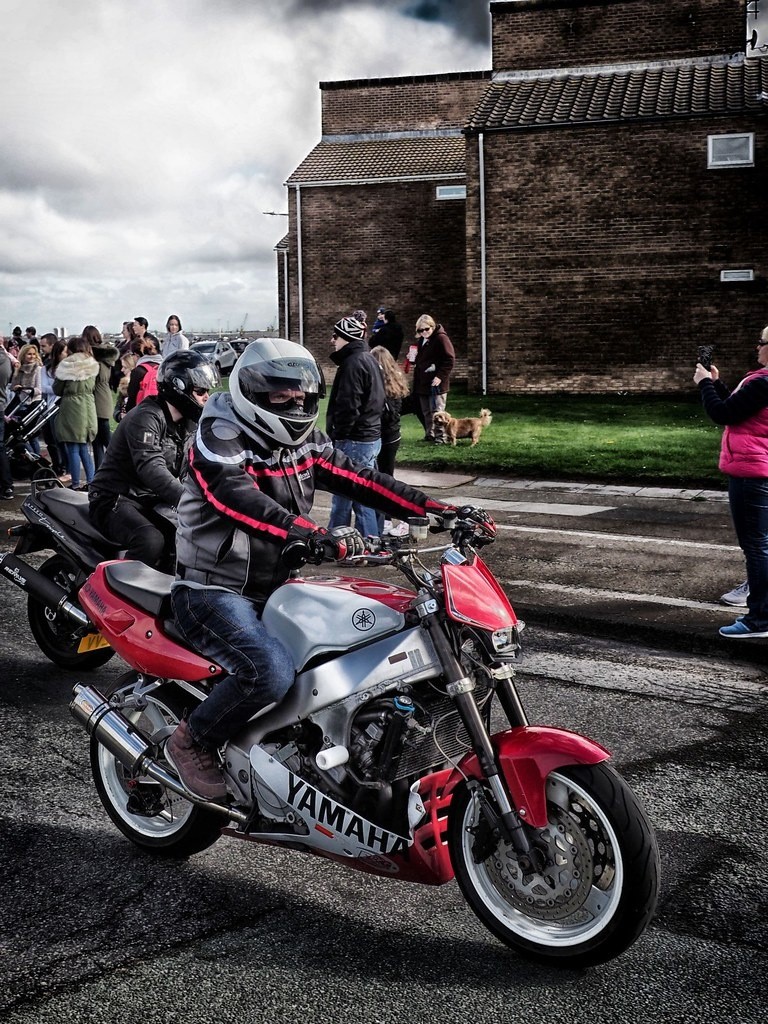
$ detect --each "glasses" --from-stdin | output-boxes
[419,328,430,332]
[759,339,768,346]
[332,333,338,340]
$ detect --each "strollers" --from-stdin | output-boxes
[1,385,60,491]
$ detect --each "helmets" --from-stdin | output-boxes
[155,350,222,423]
[229,338,326,446]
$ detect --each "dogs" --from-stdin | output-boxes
[431,407,492,447]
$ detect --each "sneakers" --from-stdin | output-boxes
[721,580,750,606]
[164,718,227,801]
[719,616,768,638]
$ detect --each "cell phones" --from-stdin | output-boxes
[698,345,711,371]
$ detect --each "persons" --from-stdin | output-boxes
[327,307,453,567]
[88,350,223,568]
[694,326,768,638]
[0,315,189,501]
[163,338,496,802]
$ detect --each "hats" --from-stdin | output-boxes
[377,308,384,313]
[334,310,367,342]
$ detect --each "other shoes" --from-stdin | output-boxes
[53,465,67,475]
[67,484,80,490]
[382,520,392,534]
[3,489,14,499]
[388,521,409,536]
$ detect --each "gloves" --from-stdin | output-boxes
[425,496,496,543]
[286,513,367,567]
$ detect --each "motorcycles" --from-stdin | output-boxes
[69,509,664,965]
[1,466,117,670]
